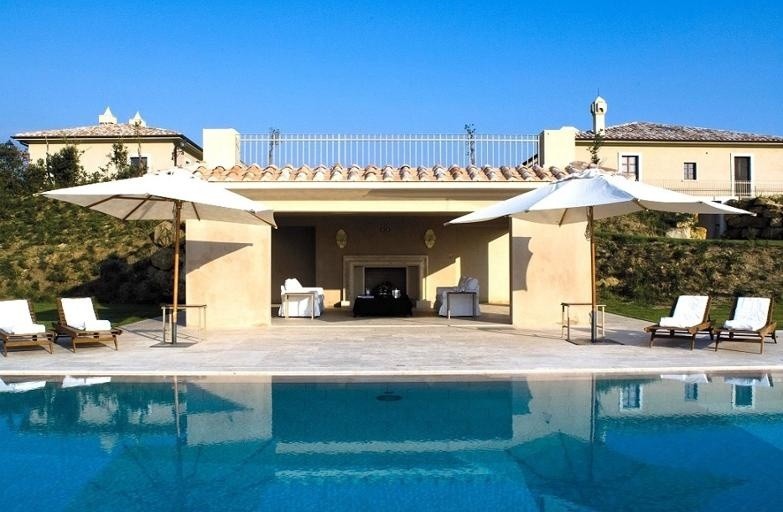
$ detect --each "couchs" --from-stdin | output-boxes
[433,275,480,319]
[277,278,325,320]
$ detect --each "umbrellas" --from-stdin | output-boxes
[443,164,759,311]
[32,165,279,322]
[75,377,280,510]
[503,379,752,511]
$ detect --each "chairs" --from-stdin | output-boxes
[645,295,778,355]
[0,377,111,396]
[0,296,122,357]
[659,372,775,387]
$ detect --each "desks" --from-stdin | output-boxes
[160,304,206,345]
[561,302,606,341]
[352,295,414,318]
